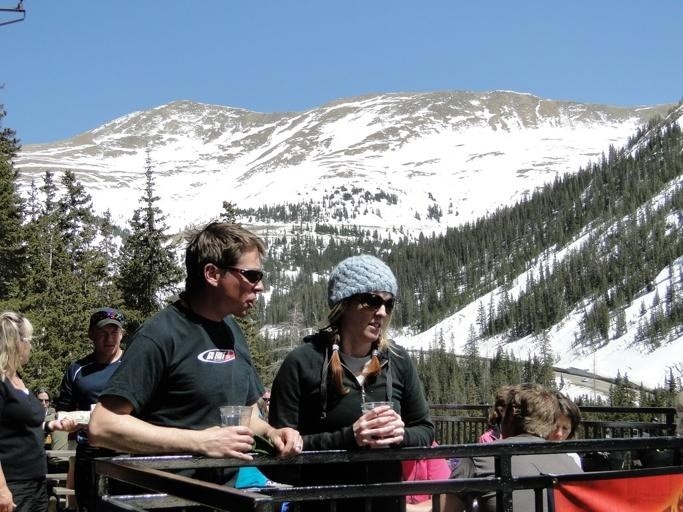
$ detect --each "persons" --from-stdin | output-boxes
[30,385,56,421]
[86,219,304,511]
[266,254,435,511]
[232,383,591,512]
[52,307,130,511]
[0,310,82,511]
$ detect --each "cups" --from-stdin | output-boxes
[219,404,253,429]
[359,400,392,449]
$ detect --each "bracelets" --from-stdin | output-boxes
[44,419,53,434]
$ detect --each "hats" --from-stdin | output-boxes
[327,255,398,308]
[88,306,126,333]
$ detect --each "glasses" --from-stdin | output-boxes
[358,293,396,314]
[224,264,264,283]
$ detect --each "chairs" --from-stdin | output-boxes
[540,467,683,512]
